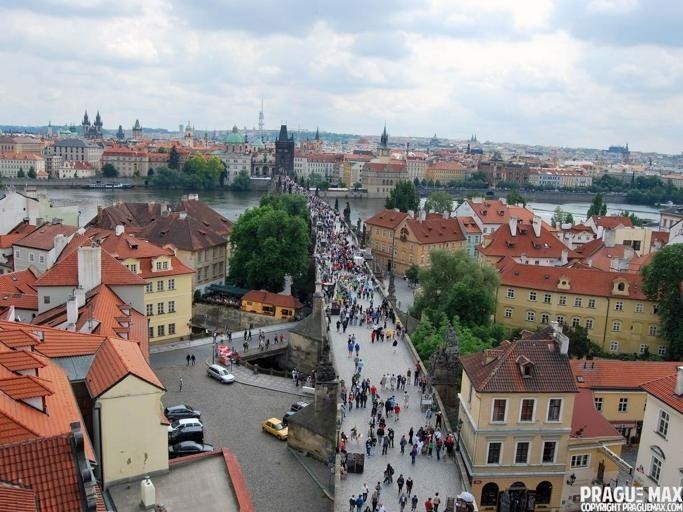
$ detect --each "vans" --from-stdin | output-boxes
[206,364,234,383]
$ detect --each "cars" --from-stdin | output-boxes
[165,403,213,456]
[261,418,288,440]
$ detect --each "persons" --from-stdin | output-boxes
[179,377,184,391]
[276,172,475,512]
[206,293,278,366]
[186,354,191,365]
[190,354,195,365]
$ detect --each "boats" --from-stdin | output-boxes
[85,179,138,191]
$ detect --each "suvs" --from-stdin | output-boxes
[282,411,297,426]
[291,399,309,412]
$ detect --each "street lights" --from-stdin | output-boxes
[404,310,409,333]
[335,419,343,454]
[456,417,464,452]
